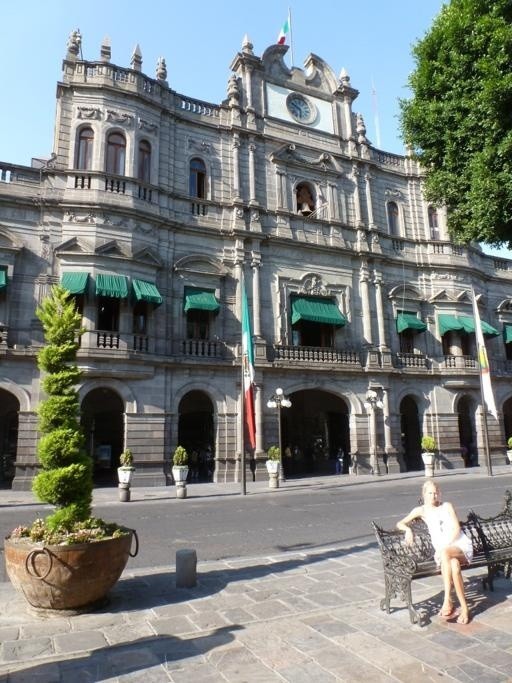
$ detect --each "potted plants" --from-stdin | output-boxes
[171,445,189,481]
[421,435,436,465]
[266,446,281,473]
[506,437,512,461]
[117,449,136,483]
[6,283,139,620]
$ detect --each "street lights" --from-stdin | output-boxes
[268,387,292,482]
[363,389,386,475]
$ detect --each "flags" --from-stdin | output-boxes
[241,269,256,452]
[277,15,291,45]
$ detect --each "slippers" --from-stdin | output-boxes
[455,610,469,624]
[438,606,454,617]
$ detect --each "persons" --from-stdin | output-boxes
[186,444,213,479]
[284,441,305,475]
[396,480,474,626]
[335,446,345,475]
[460,443,469,467]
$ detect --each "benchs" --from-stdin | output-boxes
[370,492,512,628]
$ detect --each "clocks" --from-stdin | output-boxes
[288,97,311,121]
